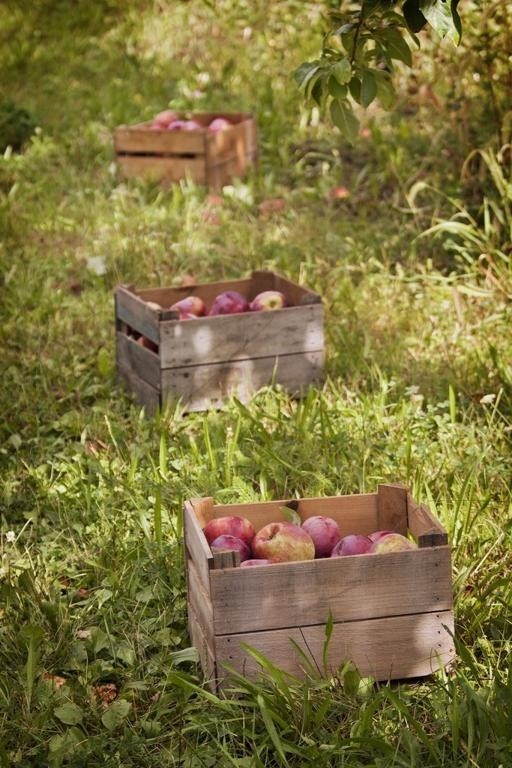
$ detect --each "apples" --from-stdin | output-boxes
[201,184,348,228]
[148,109,232,132]
[204,515,420,567]
[138,290,288,352]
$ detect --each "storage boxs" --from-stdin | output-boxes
[107,266,328,421]
[176,468,459,702]
[110,108,259,191]
